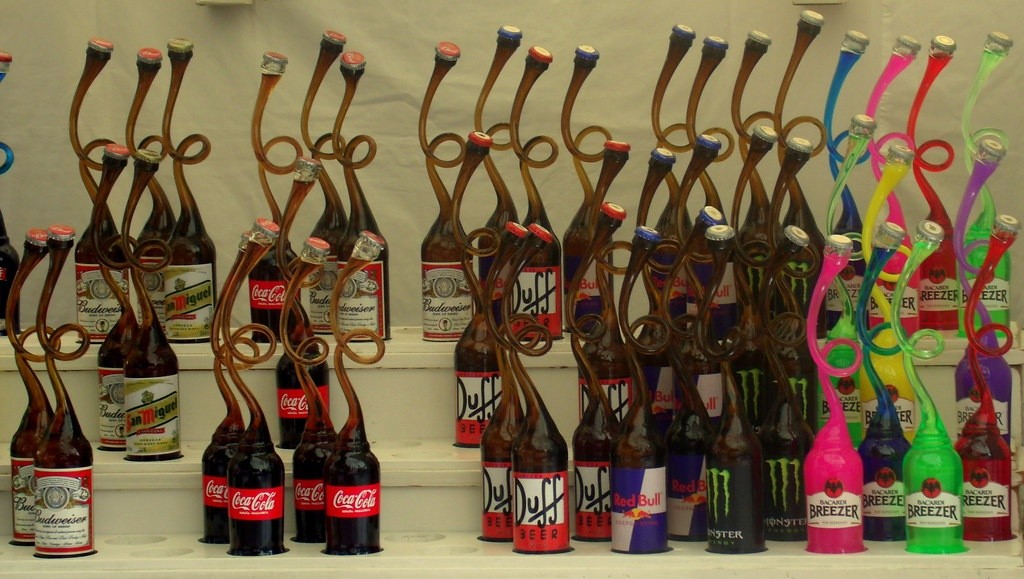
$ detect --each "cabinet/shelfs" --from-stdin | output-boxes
[0,329,1024,579]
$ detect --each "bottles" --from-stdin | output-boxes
[0,10,1018,556]
